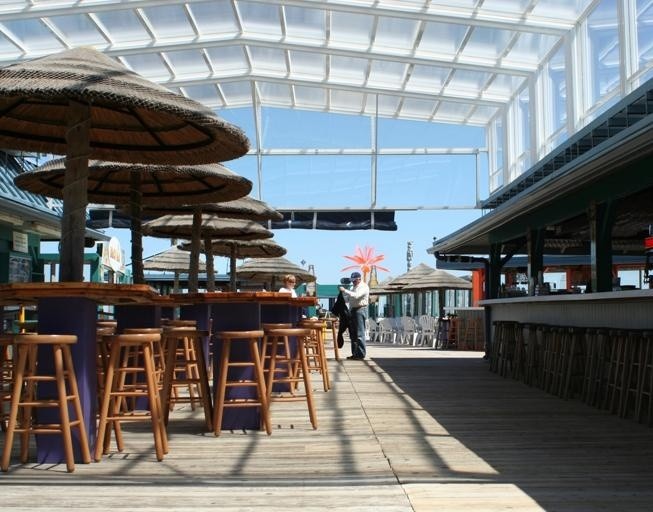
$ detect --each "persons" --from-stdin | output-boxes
[340,272,369,360]
[279,274,298,298]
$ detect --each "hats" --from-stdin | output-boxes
[349,272,361,281]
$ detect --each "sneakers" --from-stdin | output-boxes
[347,356,361,360]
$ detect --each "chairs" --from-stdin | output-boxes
[367,315,439,349]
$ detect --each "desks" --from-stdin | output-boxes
[162,292,292,432]
[443,306,485,349]
[262,296,319,392]
[1,282,163,466]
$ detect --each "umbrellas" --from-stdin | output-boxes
[14,156,253,284]
[226,256,318,291]
[177,240,288,293]
[115,198,283,291]
[142,245,217,294]
[0,45,252,280]
[369,261,473,319]
[141,215,274,293]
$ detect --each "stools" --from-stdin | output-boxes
[293,322,332,393]
[301,317,341,373]
[262,329,319,430]
[261,323,296,399]
[91,319,169,463]
[489,321,652,428]
[435,314,478,351]
[212,330,273,437]
[160,318,216,434]
[1,334,94,470]
